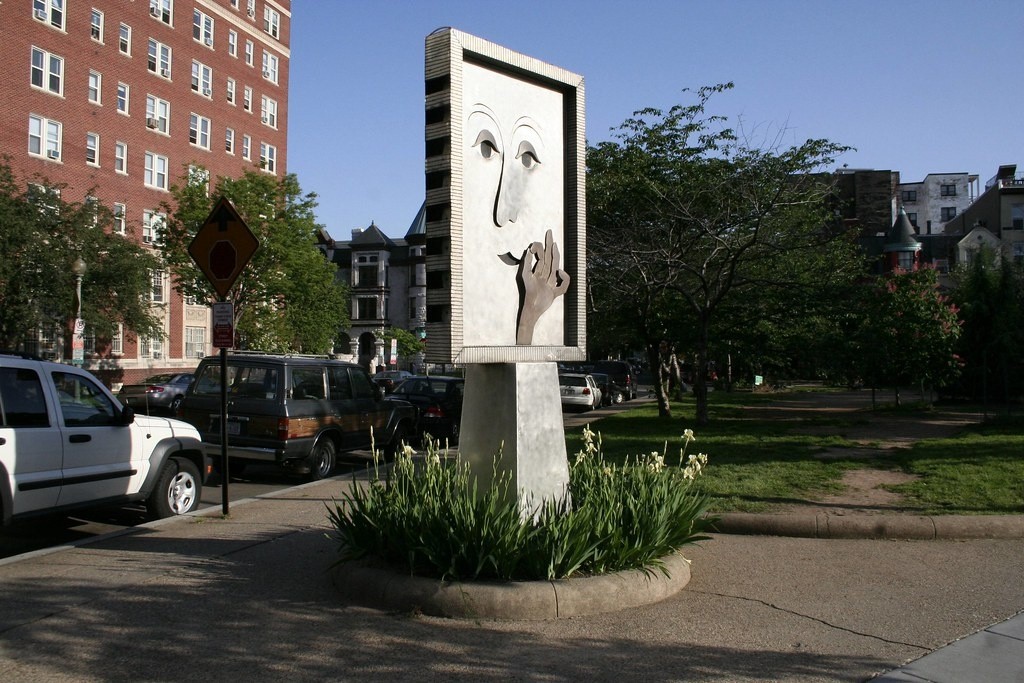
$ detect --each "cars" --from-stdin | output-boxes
[373,370,414,395]
[661,361,698,386]
[707,362,720,382]
[117,370,221,415]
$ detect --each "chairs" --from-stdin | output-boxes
[451,386,460,394]
[295,381,318,399]
[422,386,435,392]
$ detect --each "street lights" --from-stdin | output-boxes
[73,254,87,400]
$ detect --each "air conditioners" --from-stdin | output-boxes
[161,69,170,77]
[47,149,59,159]
[246,8,255,17]
[146,118,159,128]
[203,88,211,96]
[262,117,268,123]
[196,351,205,359]
[34,9,47,20]
[205,37,212,47]
[150,7,161,17]
[143,234,152,244]
[263,70,270,79]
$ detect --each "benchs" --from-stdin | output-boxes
[235,383,272,395]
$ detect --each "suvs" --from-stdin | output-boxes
[558,358,640,409]
[392,375,465,449]
[0,355,215,542]
[176,349,421,485]
